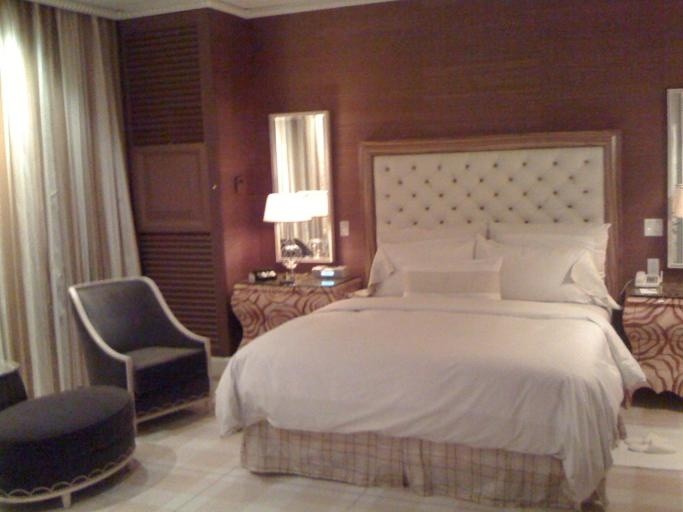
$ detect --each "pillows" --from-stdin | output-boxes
[353,220,621,310]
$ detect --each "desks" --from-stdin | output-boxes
[623,279,683,409]
[230,275,362,352]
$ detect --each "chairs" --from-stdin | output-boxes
[67,273,214,437]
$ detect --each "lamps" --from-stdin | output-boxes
[298,190,329,257]
[262,192,315,287]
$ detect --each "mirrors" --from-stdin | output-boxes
[269,110,335,265]
[665,88,683,270]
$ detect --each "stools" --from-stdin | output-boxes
[1,384,138,510]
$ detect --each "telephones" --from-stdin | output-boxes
[634,270,663,288]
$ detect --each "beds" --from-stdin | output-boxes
[239,287,615,512]
